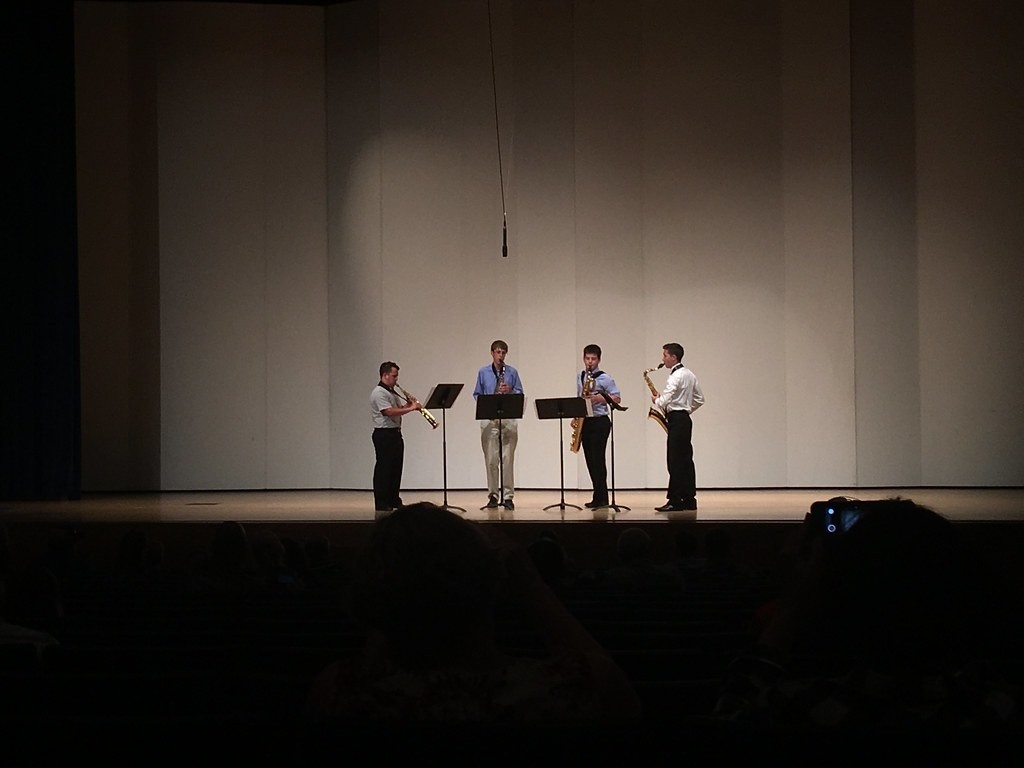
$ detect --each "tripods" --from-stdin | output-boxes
[422,383,467,512]
[475,394,524,509]
[536,398,589,511]
[590,390,631,512]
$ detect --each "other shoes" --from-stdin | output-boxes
[654,500,684,512]
[685,497,697,510]
[501,498,514,511]
[585,502,608,507]
[487,494,499,507]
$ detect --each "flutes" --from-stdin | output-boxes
[395,382,440,430]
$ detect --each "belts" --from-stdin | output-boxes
[376,428,402,432]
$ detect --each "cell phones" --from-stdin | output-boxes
[809,499,870,541]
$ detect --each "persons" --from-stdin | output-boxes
[473,340,523,510]
[570,344,621,508]
[370,361,421,511]
[651,343,705,512]
[0,498,1024,768]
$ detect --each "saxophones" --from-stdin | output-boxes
[641,362,671,437]
[568,364,593,455]
[487,358,507,428]
